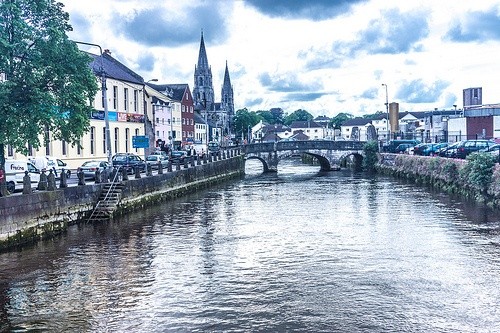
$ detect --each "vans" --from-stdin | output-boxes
[3,159,42,193]
[27,156,72,181]
[206,141,221,156]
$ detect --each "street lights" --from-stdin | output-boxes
[332,121,336,141]
[142,78,160,158]
[274,123,278,143]
[381,83,389,142]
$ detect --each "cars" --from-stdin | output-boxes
[143,150,188,169]
[76,160,109,180]
[382,139,500,161]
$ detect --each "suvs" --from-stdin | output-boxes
[113,153,148,175]
